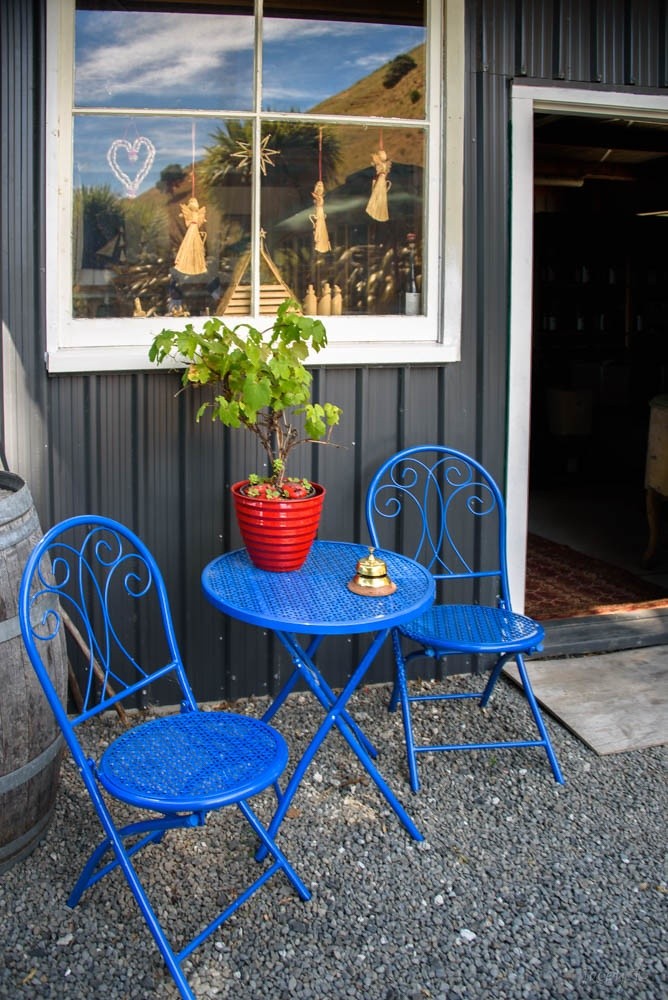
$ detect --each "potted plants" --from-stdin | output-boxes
[148,296,357,571]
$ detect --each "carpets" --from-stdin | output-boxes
[524,530,668,620]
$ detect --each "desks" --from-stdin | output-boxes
[199,538,438,860]
[643,394,668,560]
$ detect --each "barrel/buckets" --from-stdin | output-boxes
[0,470,69,876]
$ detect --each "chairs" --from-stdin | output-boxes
[366,442,566,790]
[19,515,313,1000]
